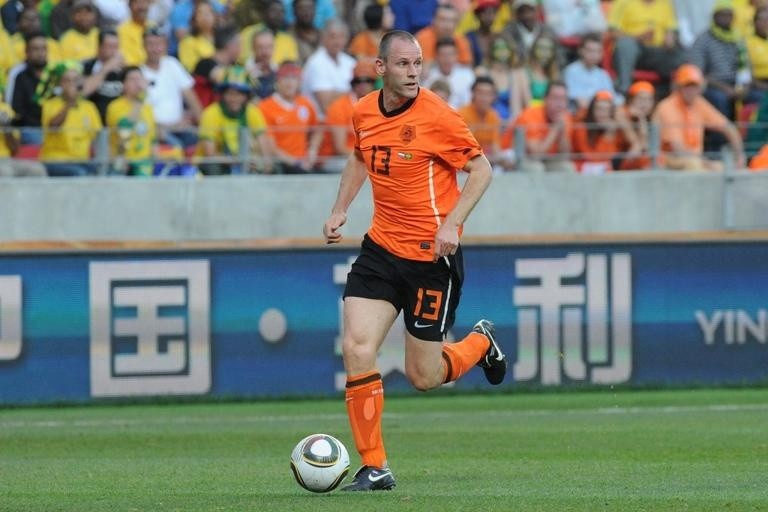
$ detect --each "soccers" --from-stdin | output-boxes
[291,434,349,492]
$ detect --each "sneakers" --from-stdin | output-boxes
[473,318,508,385]
[340,464,396,491]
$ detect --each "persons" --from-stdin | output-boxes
[462,1,767,176]
[324,30,506,490]
[0,0,502,176]
